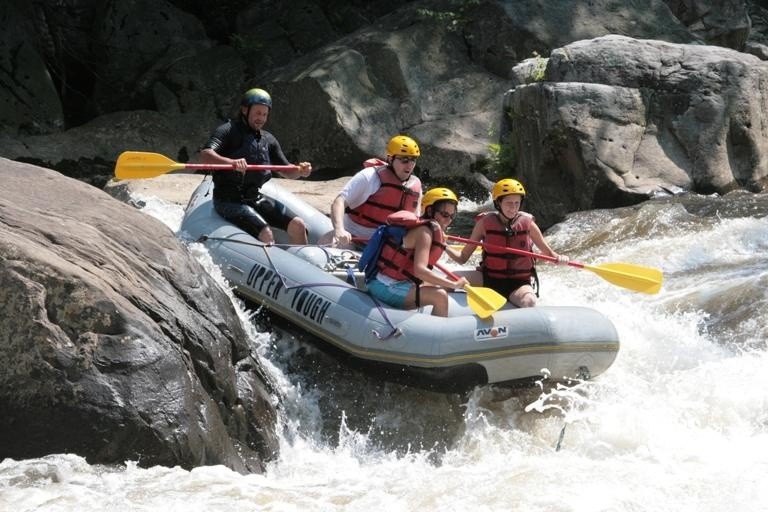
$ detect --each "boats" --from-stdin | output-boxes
[178,172,620,402]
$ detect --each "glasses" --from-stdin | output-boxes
[434,208,459,222]
[394,155,419,164]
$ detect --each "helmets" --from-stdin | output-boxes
[491,177,526,202]
[420,186,459,214]
[385,135,422,158]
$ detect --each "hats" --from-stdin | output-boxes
[241,87,274,112]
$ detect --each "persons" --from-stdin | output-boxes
[442,178,570,309]
[201,88,311,246]
[356,186,467,317]
[330,137,421,249]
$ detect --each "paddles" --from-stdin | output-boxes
[433,261,507,320]
[443,233,663,299]
[332,235,485,256]
[114,150,308,180]
